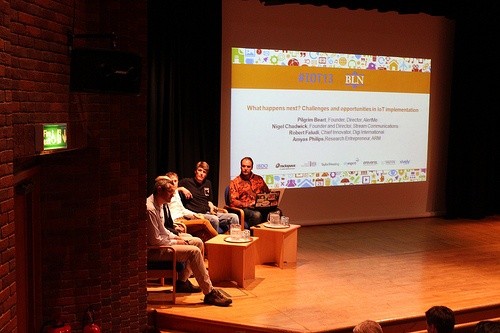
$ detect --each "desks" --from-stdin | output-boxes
[250,221,301,267]
[204,234,259,288]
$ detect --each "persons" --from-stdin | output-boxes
[228,156,283,236]
[147,160,239,306]
[352,304,500,333]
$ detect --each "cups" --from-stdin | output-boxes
[285,217,289,226]
[244,229,248,231]
[242,231,246,239]
[245,230,251,239]
[281,215,286,225]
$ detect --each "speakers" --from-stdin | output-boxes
[69,47,143,95]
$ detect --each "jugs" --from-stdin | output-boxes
[229,223,242,240]
[267,211,280,225]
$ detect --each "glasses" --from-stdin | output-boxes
[169,190,176,194]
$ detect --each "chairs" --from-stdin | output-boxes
[223,186,250,231]
[147,208,228,303]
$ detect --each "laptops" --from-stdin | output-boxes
[248,193,279,211]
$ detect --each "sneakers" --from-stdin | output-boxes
[204,289,232,305]
[176,278,202,293]
